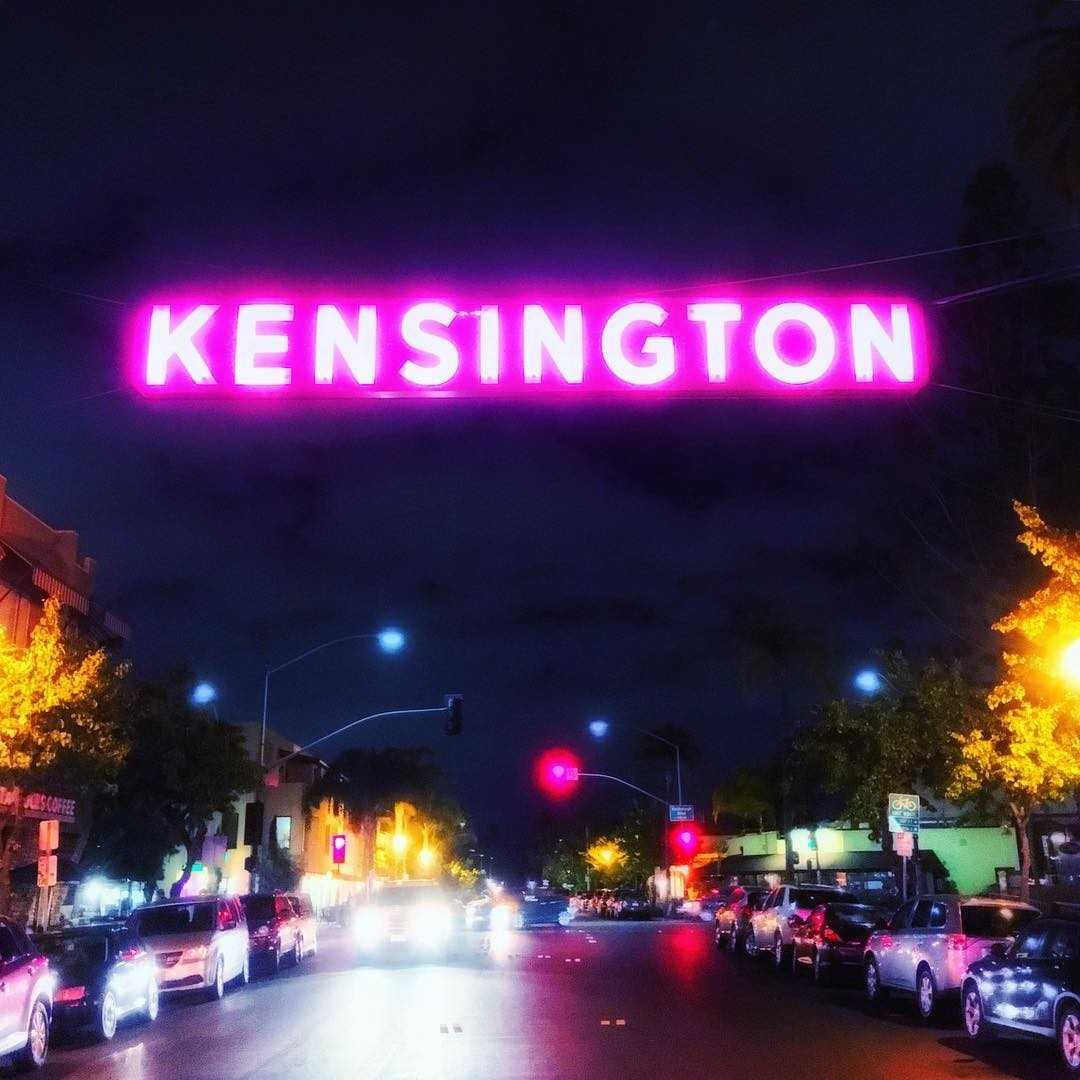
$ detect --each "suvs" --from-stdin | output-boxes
[744,881,861,973]
[127,894,252,1000]
[234,888,302,975]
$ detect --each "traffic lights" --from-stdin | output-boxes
[332,835,344,864]
[553,761,567,786]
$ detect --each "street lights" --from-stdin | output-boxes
[244,627,408,892]
[591,717,689,914]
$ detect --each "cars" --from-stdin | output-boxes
[959,917,1080,1073]
[713,885,774,956]
[790,902,888,986]
[322,879,568,962]
[287,893,319,959]
[571,889,654,919]
[0,914,60,1069]
[24,921,161,1043]
[862,894,1045,1023]
